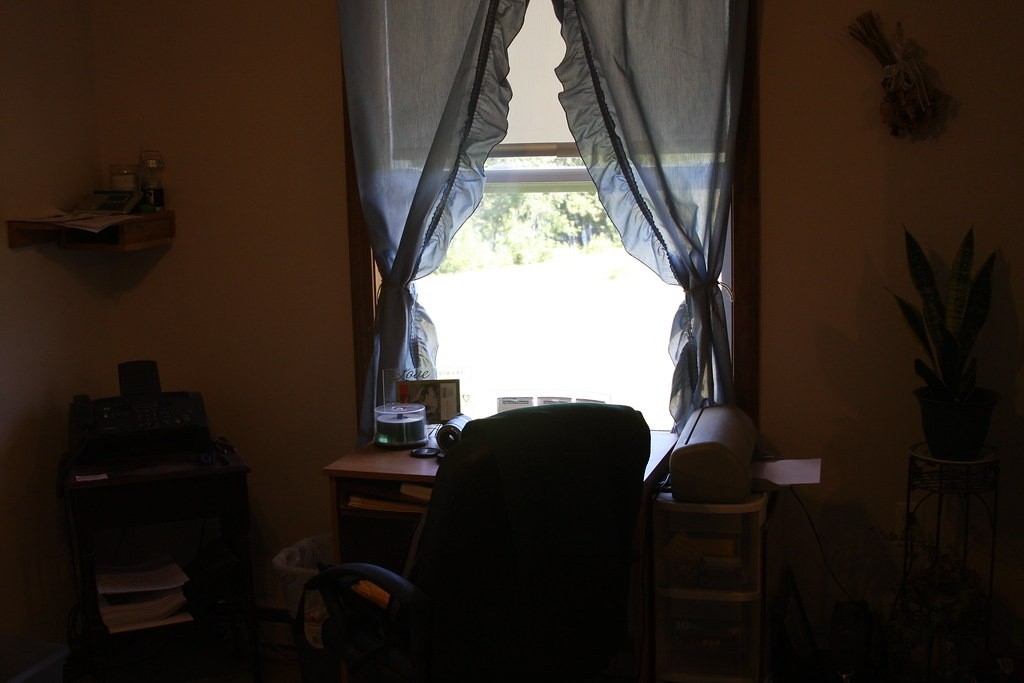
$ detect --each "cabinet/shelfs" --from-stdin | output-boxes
[647,490,781,683]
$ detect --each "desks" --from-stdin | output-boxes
[322,440,442,683]
[64,437,262,683]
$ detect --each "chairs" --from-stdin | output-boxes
[303,407,649,683]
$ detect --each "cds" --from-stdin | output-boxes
[376,414,425,442]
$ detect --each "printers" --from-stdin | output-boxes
[93,360,211,460]
[668,405,790,504]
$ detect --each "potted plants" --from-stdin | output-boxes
[882,223,1000,683]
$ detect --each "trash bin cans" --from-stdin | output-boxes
[274,534,344,683]
